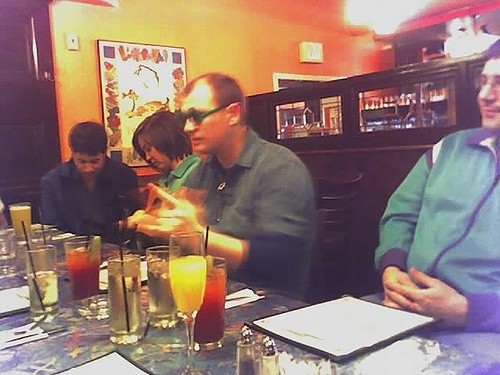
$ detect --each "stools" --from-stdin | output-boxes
[311,170,366,288]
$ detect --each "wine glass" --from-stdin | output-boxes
[169,230,212,375]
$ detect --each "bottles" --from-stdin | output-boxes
[259,337,280,375]
[235,324,258,375]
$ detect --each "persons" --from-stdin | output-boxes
[137,72,314,302]
[358,39,500,369]
[39,121,140,249]
[127,111,202,256]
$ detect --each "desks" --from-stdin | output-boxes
[0,223,500,375]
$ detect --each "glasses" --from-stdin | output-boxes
[179,104,230,125]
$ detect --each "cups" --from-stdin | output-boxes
[24,244,60,324]
[107,254,142,346]
[194,256,227,351]
[0,225,16,278]
[146,245,181,329]
[8,200,33,247]
[29,228,52,247]
[62,235,100,301]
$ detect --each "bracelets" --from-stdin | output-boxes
[204,226,209,254]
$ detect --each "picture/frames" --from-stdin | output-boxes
[97,39,188,169]
[300,42,324,64]
